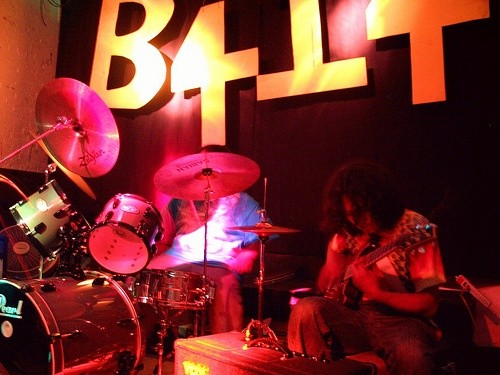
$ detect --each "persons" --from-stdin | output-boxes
[287,159,447,375]
[146,144,279,335]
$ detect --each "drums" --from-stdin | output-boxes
[10,179,90,262]
[0,270,147,375]
[131,266,213,312]
[86,193,164,275]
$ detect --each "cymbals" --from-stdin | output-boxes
[228,222,301,238]
[156,151,260,199]
[26,129,97,200]
[35,77,120,177]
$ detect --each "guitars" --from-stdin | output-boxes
[324,222,437,308]
